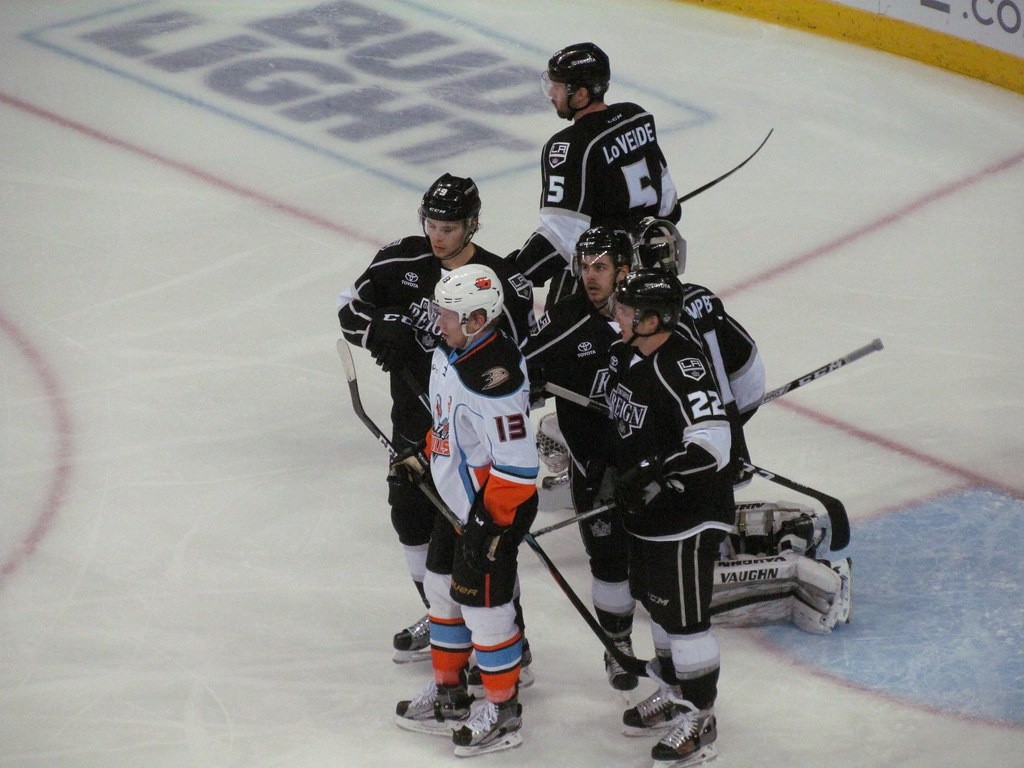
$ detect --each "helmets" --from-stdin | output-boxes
[634,217,688,274]
[421,173,481,222]
[616,269,684,330]
[433,264,504,324]
[538,413,571,457]
[578,226,633,271]
[546,43,610,96]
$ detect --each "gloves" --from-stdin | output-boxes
[525,363,547,390]
[614,453,674,516]
[503,249,544,289]
[370,345,414,372]
[459,502,510,575]
[387,439,433,486]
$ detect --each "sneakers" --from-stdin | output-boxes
[651,713,718,768]
[622,688,684,737]
[451,698,522,757]
[467,639,535,698]
[542,473,574,510]
[396,685,471,735]
[392,612,431,665]
[604,637,640,705]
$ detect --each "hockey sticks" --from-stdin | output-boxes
[677,127,777,204]
[545,382,852,552]
[762,337,885,404]
[403,364,651,677]
[337,338,466,536]
[520,494,618,540]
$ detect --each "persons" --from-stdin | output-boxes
[338,173,546,687]
[588,273,735,759]
[526,224,649,690]
[506,42,683,316]
[390,263,543,747]
[631,219,846,634]
